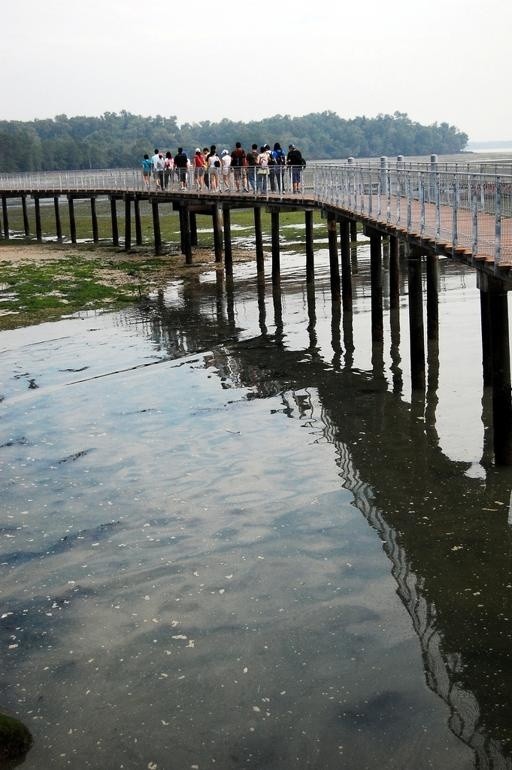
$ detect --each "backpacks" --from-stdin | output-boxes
[259,153,269,170]
[275,150,285,165]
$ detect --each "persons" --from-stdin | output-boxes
[141,142,306,194]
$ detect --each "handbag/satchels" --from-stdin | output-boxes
[214,160,220,167]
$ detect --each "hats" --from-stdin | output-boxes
[195,148,201,153]
[221,149,229,155]
[288,145,296,149]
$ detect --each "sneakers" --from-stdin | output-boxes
[145,185,301,194]
